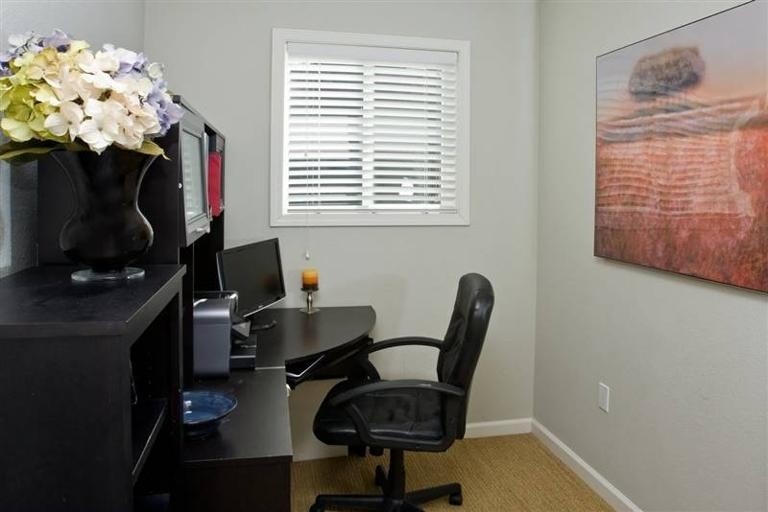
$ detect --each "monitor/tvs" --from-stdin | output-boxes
[215,237,286,332]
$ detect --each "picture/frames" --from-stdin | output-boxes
[595,1,767,296]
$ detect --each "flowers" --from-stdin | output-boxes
[1,28,189,157]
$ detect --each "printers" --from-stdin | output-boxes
[190,293,259,378]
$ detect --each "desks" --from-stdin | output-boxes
[179,307,376,511]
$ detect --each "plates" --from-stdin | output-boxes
[182,390,239,437]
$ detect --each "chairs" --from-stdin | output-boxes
[310,273,498,511]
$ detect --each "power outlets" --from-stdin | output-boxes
[597,383,610,414]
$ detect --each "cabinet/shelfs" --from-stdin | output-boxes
[15,103,226,247]
[1,261,186,512]
